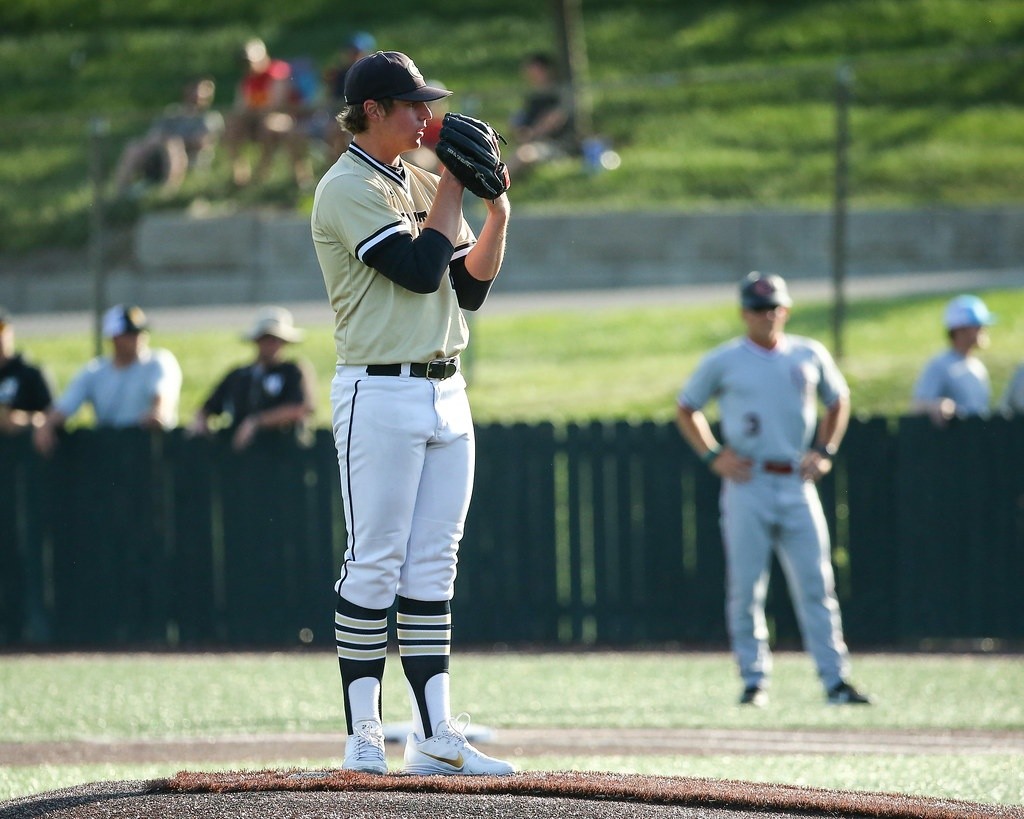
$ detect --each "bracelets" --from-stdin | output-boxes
[699,444,723,465]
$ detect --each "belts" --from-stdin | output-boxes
[764,461,791,474]
[365,360,457,380]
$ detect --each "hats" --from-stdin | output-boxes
[942,296,997,329]
[740,272,792,308]
[102,304,149,338]
[241,305,305,344]
[343,51,454,104]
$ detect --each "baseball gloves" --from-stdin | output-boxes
[435,112,512,204]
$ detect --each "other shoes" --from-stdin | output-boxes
[740,688,768,707]
[830,684,870,703]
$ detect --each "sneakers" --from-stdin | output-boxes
[341,718,389,774]
[404,713,515,775]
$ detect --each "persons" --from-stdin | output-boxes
[190,304,318,452]
[913,295,1024,422]
[0,306,64,437]
[110,32,376,203]
[675,272,871,706]
[500,53,576,176]
[35,303,183,453]
[310,52,516,778]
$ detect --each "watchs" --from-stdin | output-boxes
[815,441,839,458]
